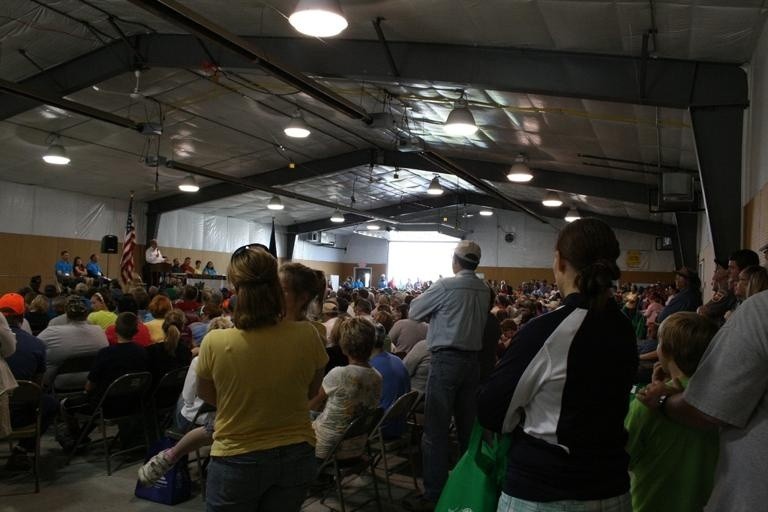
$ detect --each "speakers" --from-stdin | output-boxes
[100,233,120,254]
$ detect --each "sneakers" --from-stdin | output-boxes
[401,491,437,512]
[88,425,119,442]
[5,447,33,471]
[55,431,90,448]
[137,448,174,489]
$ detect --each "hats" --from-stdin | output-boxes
[454,241,481,263]
[714,258,729,270]
[673,269,696,278]
[322,303,339,313]
[0,293,26,316]
[368,320,385,336]
[518,301,534,312]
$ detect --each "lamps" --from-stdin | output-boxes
[426,173,444,196]
[564,202,580,222]
[287,1,348,37]
[43,136,71,165]
[541,188,563,209]
[178,172,200,192]
[330,208,346,223]
[267,194,284,210]
[284,106,311,138]
[505,155,534,183]
[443,88,478,137]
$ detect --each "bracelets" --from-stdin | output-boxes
[656,394,670,418]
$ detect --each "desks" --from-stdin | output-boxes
[178,273,228,289]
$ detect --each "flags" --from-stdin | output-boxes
[118,196,138,294]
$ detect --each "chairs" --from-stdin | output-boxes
[64,372,150,477]
[0,379,46,494]
[151,366,190,457]
[341,391,422,507]
[310,407,385,512]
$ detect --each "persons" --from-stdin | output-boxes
[634,288,768,512]
[278,261,328,348]
[623,310,722,512]
[403,339,433,414]
[144,296,175,342]
[487,277,564,353]
[86,253,111,284]
[368,323,411,427]
[55,250,76,289]
[48,294,80,328]
[35,294,110,455]
[189,303,225,354]
[86,290,117,331]
[145,309,199,372]
[105,293,153,348]
[73,256,94,283]
[306,317,394,466]
[613,244,768,363]
[171,258,184,273]
[308,271,433,344]
[0,292,62,466]
[24,294,51,338]
[17,279,123,299]
[128,286,154,324]
[194,260,203,275]
[475,218,642,512]
[180,256,195,274]
[172,285,204,313]
[136,423,214,490]
[145,239,171,293]
[405,240,490,511]
[0,312,20,470]
[202,260,217,276]
[201,286,237,319]
[173,317,231,432]
[192,245,331,512]
[60,312,148,449]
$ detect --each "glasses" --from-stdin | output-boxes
[233,243,270,258]
